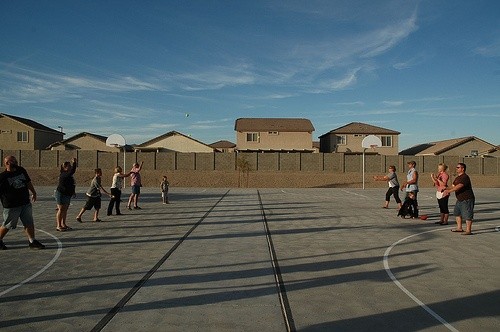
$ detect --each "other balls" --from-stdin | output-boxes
[186,113,189,117]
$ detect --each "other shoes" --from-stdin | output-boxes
[28,239,45,248]
[75,217,83,223]
[382,205,389,209]
[435,222,441,224]
[57,225,72,231]
[440,223,448,225]
[460,231,472,234]
[116,213,123,215]
[0,240,8,250]
[134,206,141,209]
[163,202,169,205]
[413,215,419,218]
[451,228,464,232]
[106,212,111,216]
[125,206,133,210]
[401,215,407,219]
[94,218,101,222]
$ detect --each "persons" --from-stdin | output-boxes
[159,176,170,204]
[373,165,402,209]
[55,158,77,232]
[78,168,110,222]
[441,163,475,235]
[430,163,451,226]
[107,166,134,216]
[397,161,418,219]
[128,160,144,210]
[0,155,46,250]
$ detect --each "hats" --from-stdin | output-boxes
[420,215,428,220]
[407,161,416,164]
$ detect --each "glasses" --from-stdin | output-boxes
[456,167,464,168]
[388,168,392,169]
